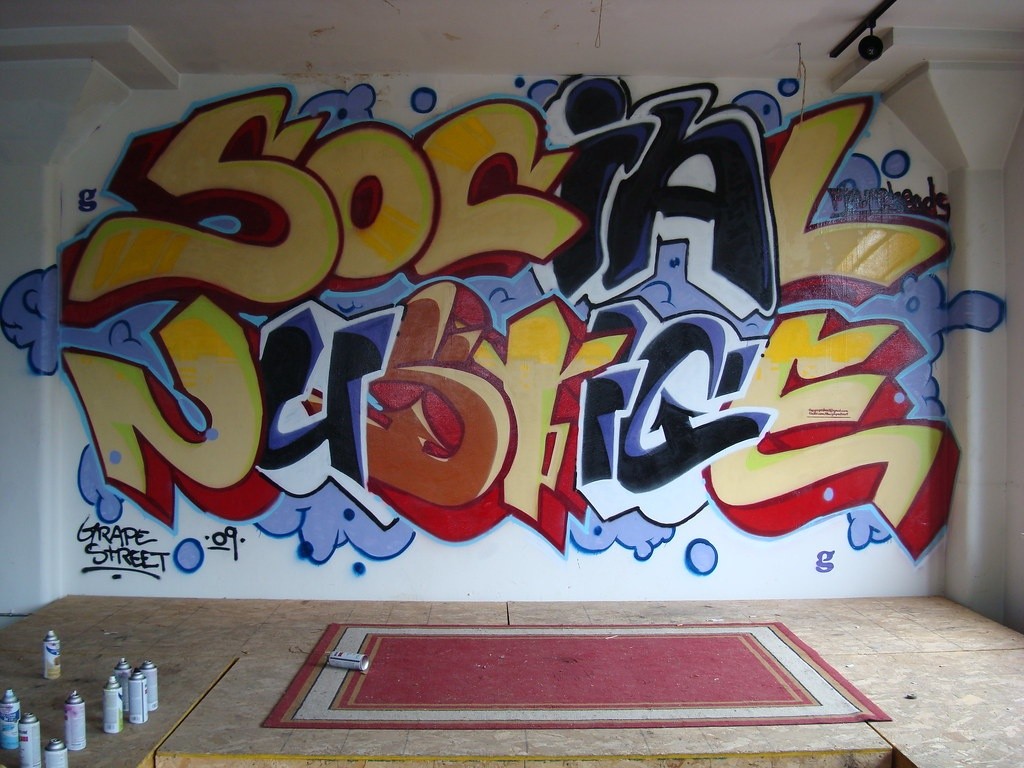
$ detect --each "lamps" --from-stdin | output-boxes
[857,18,884,61]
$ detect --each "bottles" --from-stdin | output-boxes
[42,631,60,679]
[140,661,158,712]
[18,712,42,768]
[44,738,69,768]
[102,676,123,733]
[325,652,369,670]
[64,690,86,751]
[128,668,148,723]
[114,658,133,712]
[0,688,21,750]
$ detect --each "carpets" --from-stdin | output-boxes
[261,617,893,731]
